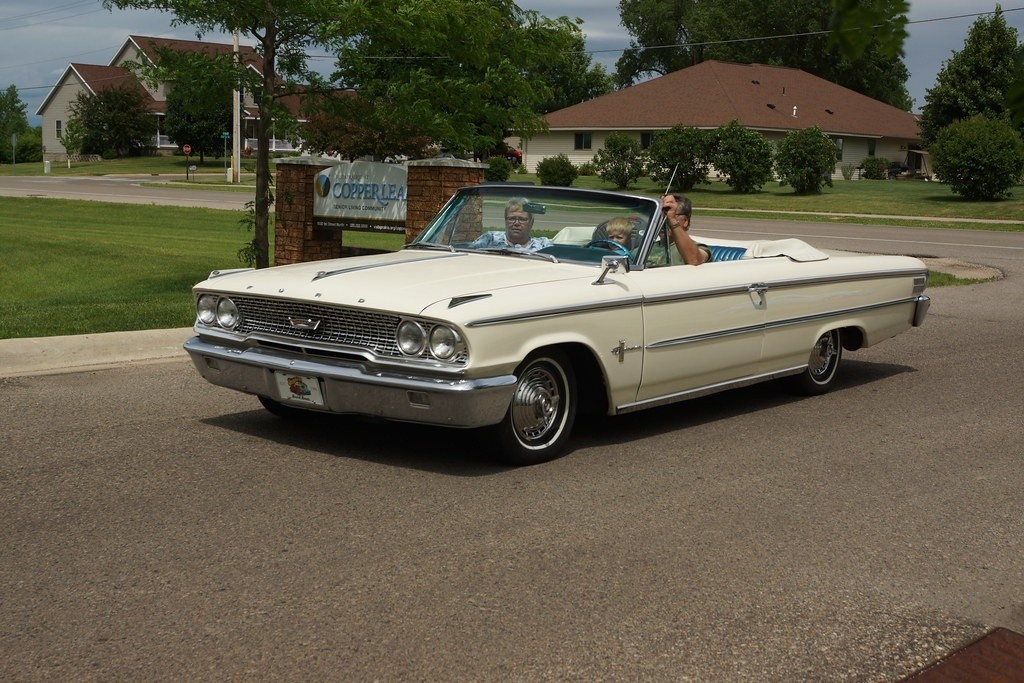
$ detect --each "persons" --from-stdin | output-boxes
[465,195,553,256]
[630,194,713,266]
[607,218,633,249]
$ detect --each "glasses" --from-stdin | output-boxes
[505,216,532,224]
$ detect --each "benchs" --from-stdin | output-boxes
[707,246,746,261]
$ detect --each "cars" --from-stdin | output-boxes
[385,154,414,163]
[502,146,522,162]
[181,182,932,462]
[434,150,465,160]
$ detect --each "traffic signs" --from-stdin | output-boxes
[220,131,231,140]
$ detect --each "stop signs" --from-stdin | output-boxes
[183,145,191,154]
